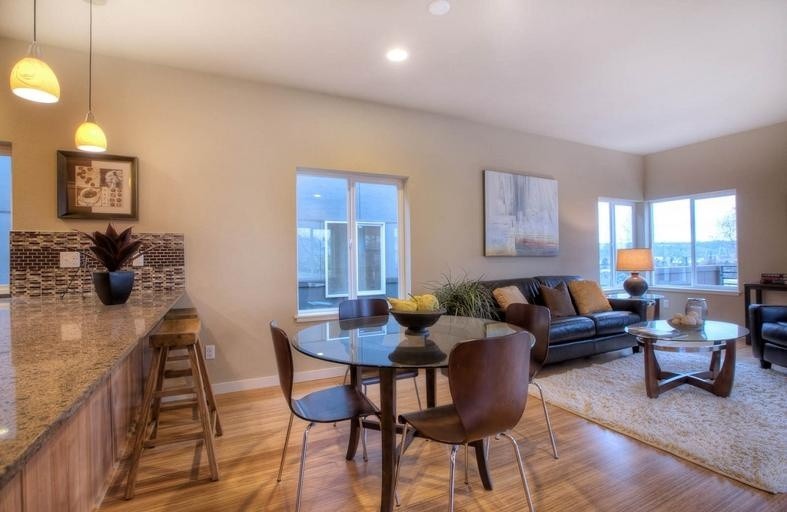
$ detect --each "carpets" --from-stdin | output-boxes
[528,337,787,495]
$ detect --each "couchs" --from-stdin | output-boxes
[748,304,787,369]
[474,275,647,365]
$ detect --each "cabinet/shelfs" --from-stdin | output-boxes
[744,282,787,345]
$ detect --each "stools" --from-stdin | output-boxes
[124,318,223,500]
[160,307,201,405]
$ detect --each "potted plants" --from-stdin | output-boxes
[421,265,502,378]
[59,223,165,305]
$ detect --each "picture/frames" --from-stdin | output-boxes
[56,150,138,221]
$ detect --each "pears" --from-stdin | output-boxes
[384,294,418,312]
[408,293,422,305]
[418,294,441,312]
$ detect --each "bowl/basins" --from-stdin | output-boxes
[668,318,702,330]
[388,336,447,365]
[389,308,447,335]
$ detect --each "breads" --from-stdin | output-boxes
[672,311,704,326]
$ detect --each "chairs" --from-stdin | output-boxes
[339,298,423,411]
[394,330,535,512]
[269,319,401,512]
[486,303,560,460]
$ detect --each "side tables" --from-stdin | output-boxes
[606,293,665,343]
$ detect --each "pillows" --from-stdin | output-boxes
[568,279,614,316]
[539,280,576,317]
[492,286,530,312]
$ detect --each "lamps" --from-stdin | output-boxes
[74,0,108,152]
[615,247,653,296]
[10,0,61,104]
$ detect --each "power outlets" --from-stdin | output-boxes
[60,252,80,268]
[206,345,216,360]
